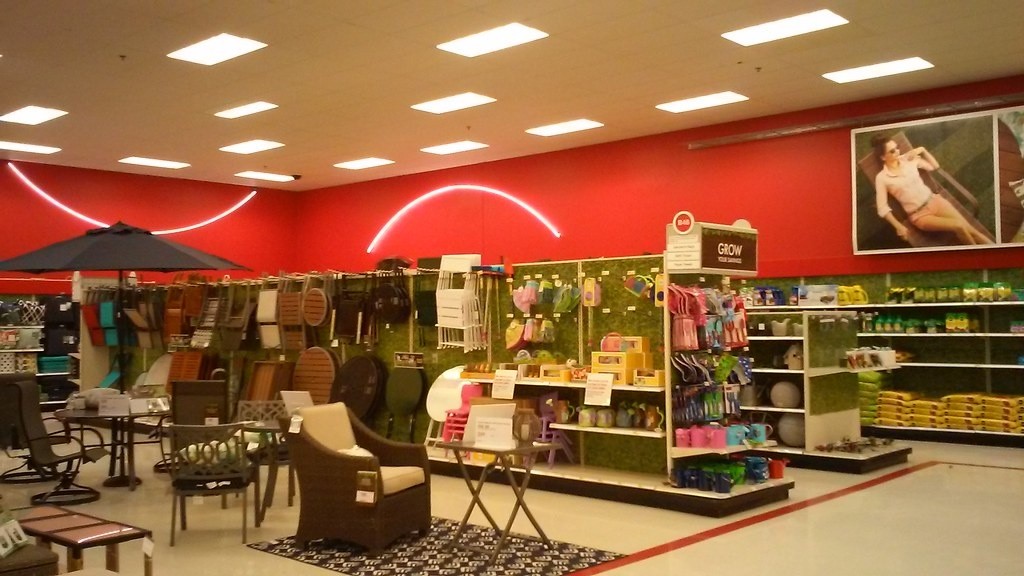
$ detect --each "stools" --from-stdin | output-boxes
[372,270,411,322]
[302,272,331,328]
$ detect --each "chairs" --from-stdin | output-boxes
[435,270,487,353]
[97,353,131,389]
[278,402,431,558]
[171,382,239,499]
[414,272,438,347]
[442,384,485,459]
[82,270,316,353]
[123,373,148,394]
[329,272,380,346]
[857,131,996,248]
[538,391,576,470]
[0,371,111,506]
[169,423,261,547]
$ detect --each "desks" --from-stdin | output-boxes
[385,366,425,444]
[166,352,224,396]
[424,365,471,450]
[331,356,387,428]
[0,503,153,576]
[53,408,172,492]
[144,354,172,384]
[438,441,563,565]
[244,360,294,402]
[292,347,342,406]
[245,420,295,520]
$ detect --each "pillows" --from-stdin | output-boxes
[336,445,375,459]
[180,421,272,467]
[65,387,121,410]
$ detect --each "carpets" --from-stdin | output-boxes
[247,517,629,576]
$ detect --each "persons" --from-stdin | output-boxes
[870,133,995,247]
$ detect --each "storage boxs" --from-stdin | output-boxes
[38,356,71,373]
[472,397,536,440]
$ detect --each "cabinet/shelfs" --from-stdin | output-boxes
[465,378,666,438]
[0,325,76,412]
[669,440,795,519]
[743,311,912,473]
[746,301,1024,447]
[67,352,80,385]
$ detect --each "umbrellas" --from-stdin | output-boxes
[1,221,254,488]
